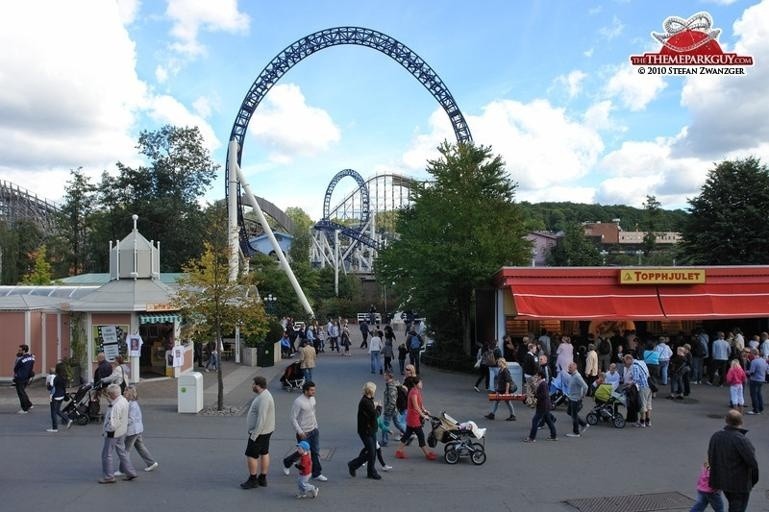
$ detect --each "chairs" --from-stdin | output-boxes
[386,313,426,322]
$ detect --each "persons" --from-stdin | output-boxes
[240,305,769,512]
[193,329,224,372]
[14,345,35,414]
[131,339,140,350]
[46,350,159,482]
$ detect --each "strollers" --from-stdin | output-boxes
[54,382,107,428]
[586,383,631,428]
[422,408,489,466]
[279,359,309,392]
[546,380,570,411]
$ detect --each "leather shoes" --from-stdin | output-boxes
[367,473,381,480]
[348,461,356,477]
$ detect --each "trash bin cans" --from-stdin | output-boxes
[177,372,204,414]
[258,345,276,368]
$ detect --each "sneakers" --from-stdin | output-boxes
[396,450,408,458]
[378,432,417,447]
[473,386,480,392]
[505,415,516,421]
[425,452,437,460]
[45,429,59,433]
[239,457,327,499]
[747,410,760,415]
[484,413,494,419]
[522,417,590,443]
[632,421,651,428]
[66,420,73,429]
[382,465,392,470]
[652,391,689,400]
[17,410,27,414]
[97,462,159,484]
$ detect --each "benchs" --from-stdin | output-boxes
[202,341,234,361]
[356,313,382,325]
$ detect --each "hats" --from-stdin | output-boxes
[296,441,310,450]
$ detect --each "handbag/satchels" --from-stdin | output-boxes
[474,356,482,368]
[119,366,126,395]
[509,382,517,394]
[647,376,658,392]
[426,416,441,448]
